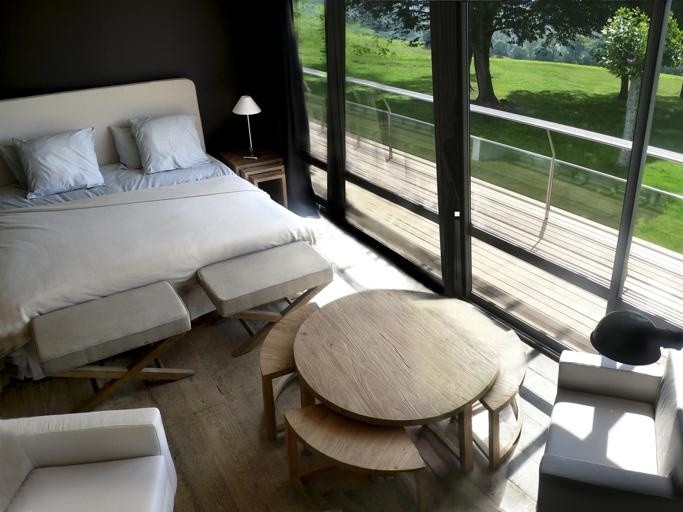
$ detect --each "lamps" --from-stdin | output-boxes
[590,308,683,366]
[233,96,261,159]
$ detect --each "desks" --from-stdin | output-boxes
[259,286,527,512]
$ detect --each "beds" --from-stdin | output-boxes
[0,79,319,398]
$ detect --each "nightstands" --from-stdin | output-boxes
[222,150,288,208]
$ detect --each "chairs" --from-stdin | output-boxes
[538,350,683,500]
[0,407,178,512]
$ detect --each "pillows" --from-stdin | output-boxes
[130,112,208,174]
[10,128,105,198]
[108,125,142,170]
[0,143,28,189]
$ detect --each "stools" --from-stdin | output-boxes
[28,280,195,413]
[196,240,333,357]
